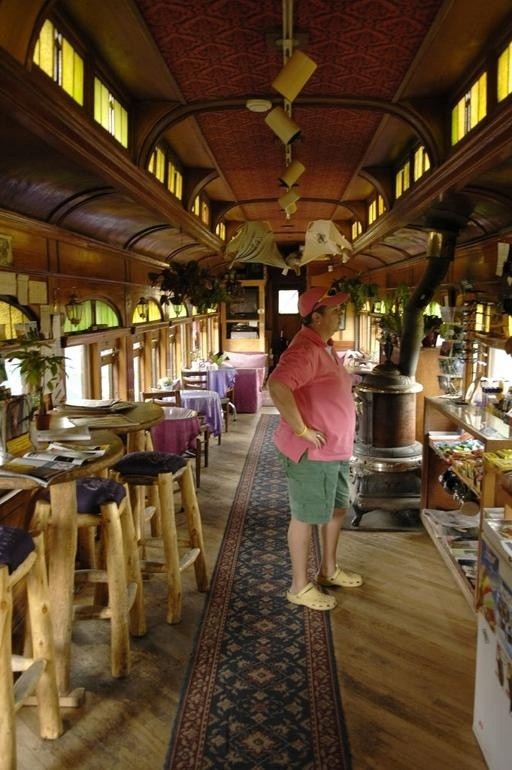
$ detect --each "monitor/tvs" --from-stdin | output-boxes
[227,286,259,319]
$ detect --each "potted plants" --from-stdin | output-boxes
[4,327,72,430]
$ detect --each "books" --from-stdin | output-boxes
[0,391,141,490]
[422,503,507,593]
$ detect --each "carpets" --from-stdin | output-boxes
[163,414,354,768]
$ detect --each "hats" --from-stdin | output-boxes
[298,287,349,317]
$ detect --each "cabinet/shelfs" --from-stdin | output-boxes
[419,393,512,681]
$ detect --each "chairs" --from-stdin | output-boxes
[142,361,236,488]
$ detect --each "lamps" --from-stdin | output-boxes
[221,220,354,272]
[127,296,149,319]
[52,285,84,328]
[264,50,318,211]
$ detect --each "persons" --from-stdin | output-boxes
[265,285,364,612]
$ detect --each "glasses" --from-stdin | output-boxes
[318,287,338,304]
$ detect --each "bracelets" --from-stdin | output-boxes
[295,424,307,437]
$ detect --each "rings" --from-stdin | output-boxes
[316,434,321,438]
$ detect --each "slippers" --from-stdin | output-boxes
[315,565,363,589]
[287,583,337,610]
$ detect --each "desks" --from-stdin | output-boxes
[1,398,165,588]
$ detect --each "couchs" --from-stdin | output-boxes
[215,351,268,413]
